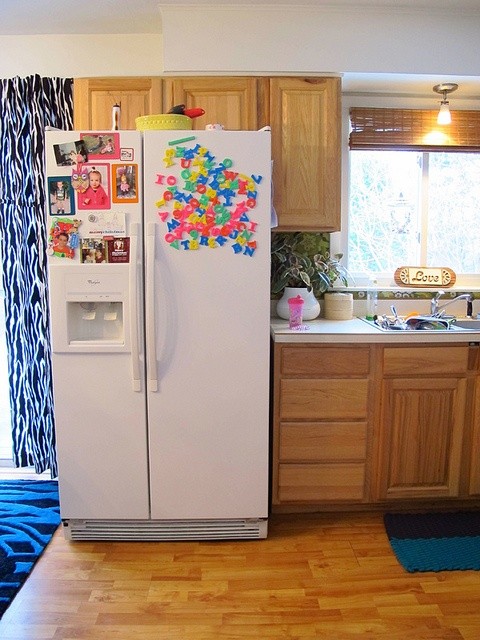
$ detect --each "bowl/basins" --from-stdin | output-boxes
[135,114,192,130]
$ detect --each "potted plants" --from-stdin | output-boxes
[271,231,351,321]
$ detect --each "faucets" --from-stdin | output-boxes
[429,290,472,313]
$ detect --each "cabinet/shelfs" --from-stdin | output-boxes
[271,348,373,519]
[262,75,340,232]
[374,347,479,509]
[73,80,256,131]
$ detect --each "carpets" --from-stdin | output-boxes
[384,509,480,574]
[1,476,62,616]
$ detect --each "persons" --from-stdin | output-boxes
[55,180,65,212]
[54,234,73,255]
[102,140,114,154]
[82,238,106,263]
[78,166,107,209]
[88,137,104,152]
[69,150,78,165]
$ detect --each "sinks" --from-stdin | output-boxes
[372,314,457,332]
[453,320,480,330]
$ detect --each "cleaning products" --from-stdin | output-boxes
[367,279,378,321]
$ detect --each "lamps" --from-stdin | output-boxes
[435,90,451,122]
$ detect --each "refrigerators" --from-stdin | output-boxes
[43,126,273,542]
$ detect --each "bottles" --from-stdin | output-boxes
[366,271,378,321]
[287,294,304,328]
